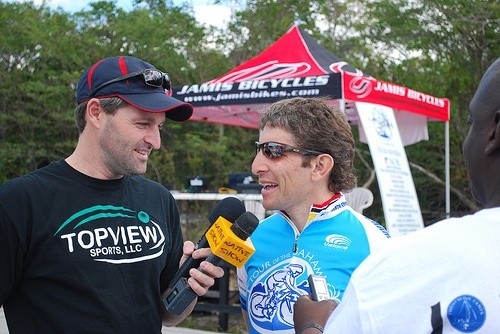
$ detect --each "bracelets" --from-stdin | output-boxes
[296,323,324,334]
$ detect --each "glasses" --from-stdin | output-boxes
[255,141,322,159]
[88,69,171,97]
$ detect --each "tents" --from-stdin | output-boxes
[166,25,450,221]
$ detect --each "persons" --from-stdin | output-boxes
[293,58,500,334]
[0,56,224,334]
[237,98,391,334]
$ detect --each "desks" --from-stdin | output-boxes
[169,191,264,248]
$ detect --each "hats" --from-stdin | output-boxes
[78,56,193,121]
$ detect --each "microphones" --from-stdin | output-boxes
[163,212,260,316]
[168,197,246,289]
